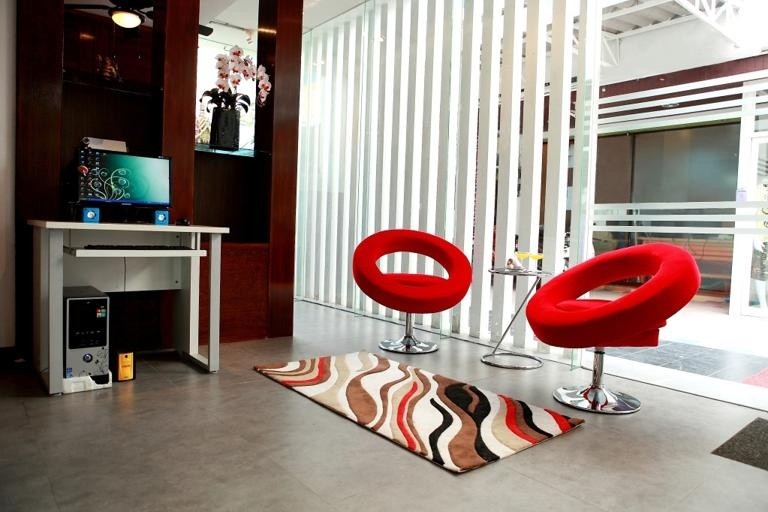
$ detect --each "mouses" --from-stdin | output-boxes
[176,218,191,226]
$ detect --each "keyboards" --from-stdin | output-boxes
[84,245,192,250]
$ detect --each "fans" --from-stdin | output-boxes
[64,0,213,37]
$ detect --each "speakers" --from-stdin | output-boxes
[82,208,100,223]
[153,209,169,225]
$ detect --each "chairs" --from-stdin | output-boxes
[350,226,475,358]
[524,241,700,417]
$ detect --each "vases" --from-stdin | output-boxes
[208,106,243,151]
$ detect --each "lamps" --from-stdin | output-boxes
[107,5,145,29]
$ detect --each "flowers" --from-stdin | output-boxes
[198,42,273,117]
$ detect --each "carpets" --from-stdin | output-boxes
[249,350,587,476]
[706,413,766,469]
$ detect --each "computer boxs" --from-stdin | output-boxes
[63,286,109,384]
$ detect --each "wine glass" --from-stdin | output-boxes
[515,242,544,271]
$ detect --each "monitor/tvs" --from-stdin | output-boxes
[74,147,173,209]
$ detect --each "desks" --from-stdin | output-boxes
[481,264,551,374]
[23,217,231,397]
